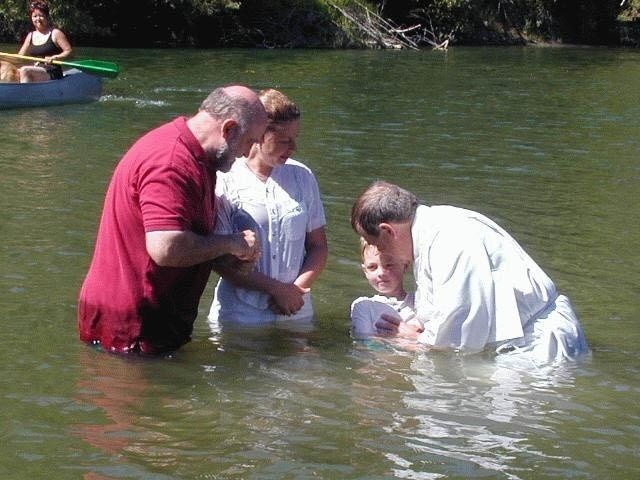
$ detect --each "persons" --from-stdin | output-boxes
[349,234,425,354]
[76,84,269,356]
[351,182,588,368]
[211,89,329,331]
[4,0,72,83]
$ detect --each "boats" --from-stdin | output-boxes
[0,68,103,108]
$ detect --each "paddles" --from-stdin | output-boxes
[0,51,120,80]
